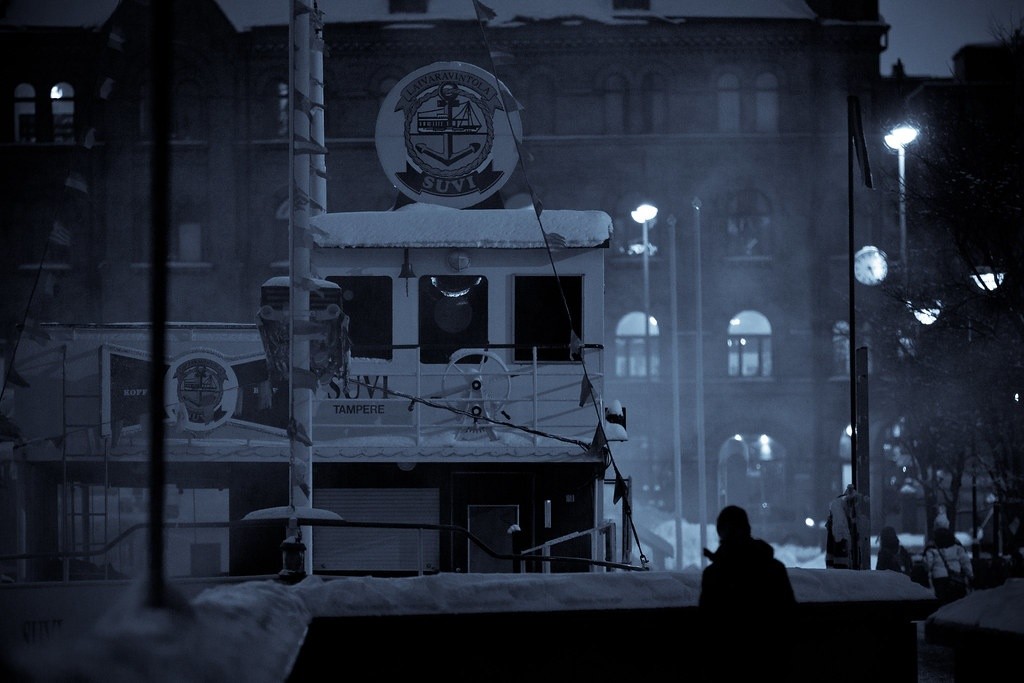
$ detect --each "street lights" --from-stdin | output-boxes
[881,123,923,377]
[630,202,659,570]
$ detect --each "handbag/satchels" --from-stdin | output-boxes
[948,570,967,594]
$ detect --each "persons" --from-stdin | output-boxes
[696,506,796,607]
[874,527,913,575]
[924,528,974,598]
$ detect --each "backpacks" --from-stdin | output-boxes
[882,544,909,577]
